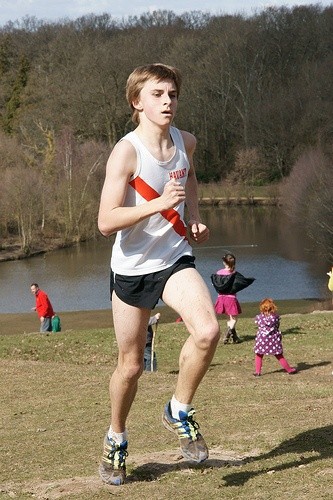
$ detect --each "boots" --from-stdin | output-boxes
[223,328,243,344]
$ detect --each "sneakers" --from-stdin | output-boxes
[162,401,209,463]
[99,431,129,486]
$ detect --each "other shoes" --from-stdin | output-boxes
[289,368,298,374]
[252,373,261,376]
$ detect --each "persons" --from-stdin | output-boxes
[211,253,256,345]
[253,298,297,377]
[143,312,161,370]
[97,65,220,485]
[327,267,333,292]
[30,283,62,333]
